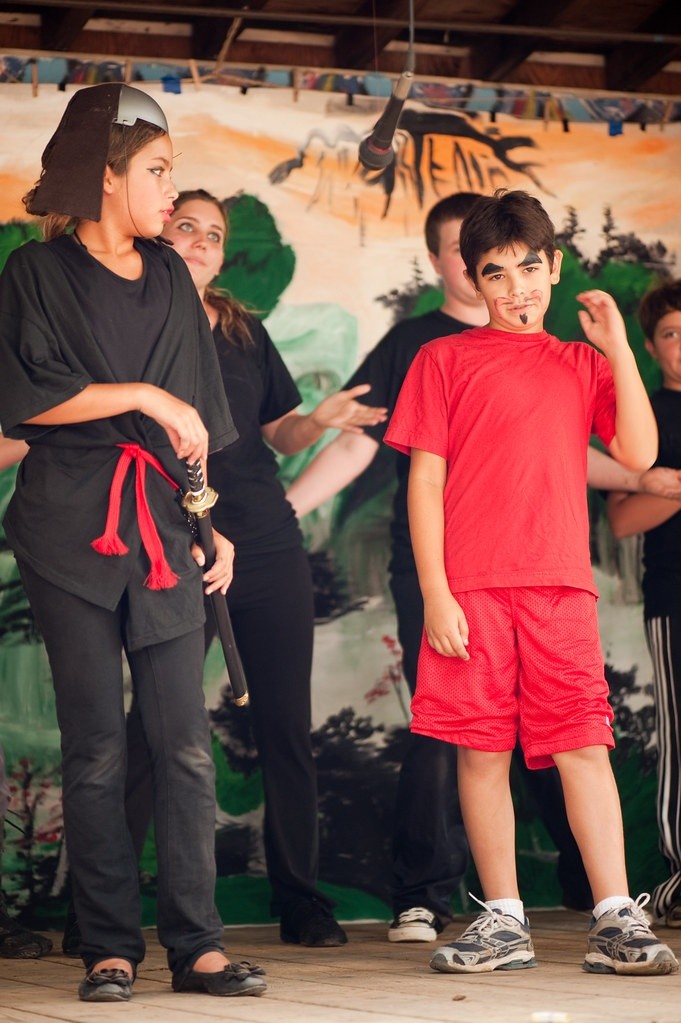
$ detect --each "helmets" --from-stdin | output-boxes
[28,82,168,221]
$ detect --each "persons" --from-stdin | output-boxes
[382,188,681,976]
[287,192,681,942]
[0,83,267,1002]
[0,189,388,948]
[603,280,681,926]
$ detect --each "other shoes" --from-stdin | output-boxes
[665,909,681,927]
[78,969,131,1001]
[172,958,267,996]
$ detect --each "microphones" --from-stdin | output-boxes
[358,71,413,171]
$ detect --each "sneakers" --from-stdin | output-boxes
[429,892,539,973]
[62,900,82,958]
[387,905,439,941]
[278,899,349,946]
[582,893,679,975]
